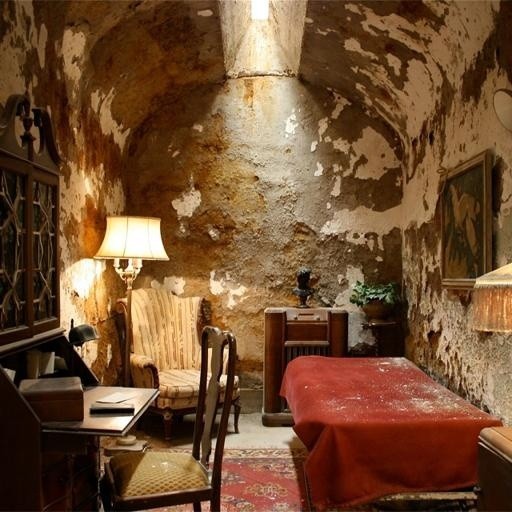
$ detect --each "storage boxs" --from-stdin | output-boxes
[19,377,84,422]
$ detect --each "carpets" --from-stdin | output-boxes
[108,447,379,512]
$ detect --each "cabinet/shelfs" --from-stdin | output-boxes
[262,306,349,427]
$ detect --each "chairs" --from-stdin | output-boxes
[113,287,241,442]
[99,325,237,512]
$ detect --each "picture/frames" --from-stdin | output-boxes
[440,149,496,290]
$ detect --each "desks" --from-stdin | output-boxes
[0,387,161,511]
[279,355,504,512]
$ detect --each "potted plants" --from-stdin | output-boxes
[350,278,401,321]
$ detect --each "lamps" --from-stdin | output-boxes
[471,260,511,339]
[93,216,169,386]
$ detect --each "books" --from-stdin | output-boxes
[88,403,136,415]
[95,391,138,404]
